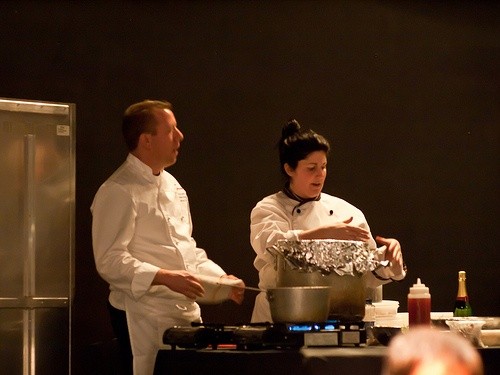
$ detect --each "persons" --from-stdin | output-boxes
[383,322,483,375]
[250,120,407,326]
[90,100,246,375]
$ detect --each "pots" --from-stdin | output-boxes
[266,287,331,323]
[265,240,367,323]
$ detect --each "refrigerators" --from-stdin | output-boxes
[0,101,76,375]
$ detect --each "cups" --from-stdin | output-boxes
[445,321,485,347]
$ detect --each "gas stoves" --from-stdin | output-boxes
[163,323,368,350]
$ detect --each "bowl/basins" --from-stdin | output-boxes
[431,319,454,330]
[481,330,500,345]
[371,326,401,346]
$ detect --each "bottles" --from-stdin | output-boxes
[453,270,472,317]
[408,278,431,332]
